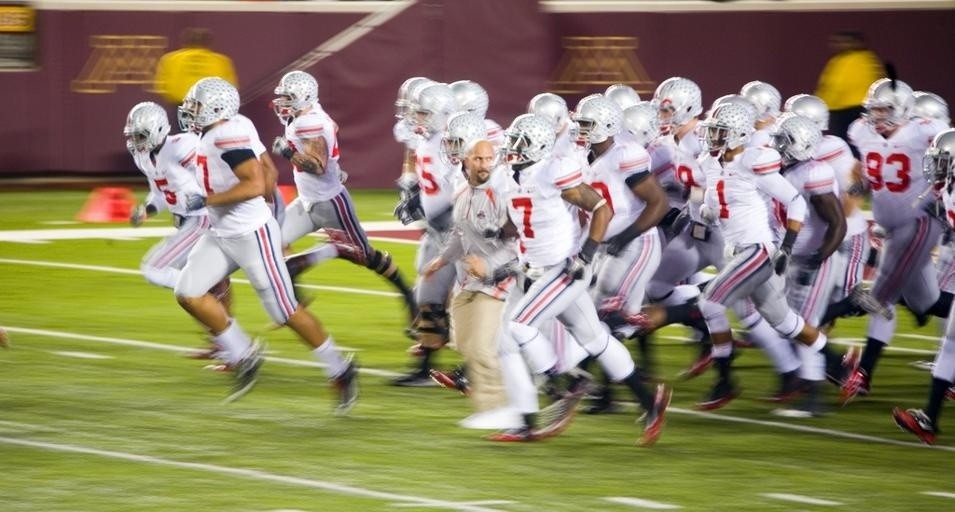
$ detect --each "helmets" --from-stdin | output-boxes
[393,77,504,163]
[125,77,241,156]
[697,81,831,163]
[860,77,954,188]
[270,72,318,125]
[501,77,703,166]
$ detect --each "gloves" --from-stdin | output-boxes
[185,193,205,211]
[392,176,423,225]
[129,204,146,225]
[603,227,639,255]
[563,251,590,284]
[771,246,825,287]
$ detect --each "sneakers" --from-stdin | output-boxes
[430,362,469,394]
[894,403,937,445]
[636,384,672,446]
[333,348,359,413]
[673,341,871,413]
[182,348,266,405]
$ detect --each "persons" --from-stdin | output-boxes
[824,78,955,446]
[393,77,863,449]
[153,27,240,135]
[124,100,233,360]
[813,28,886,160]
[173,77,360,418]
[266,70,428,356]
